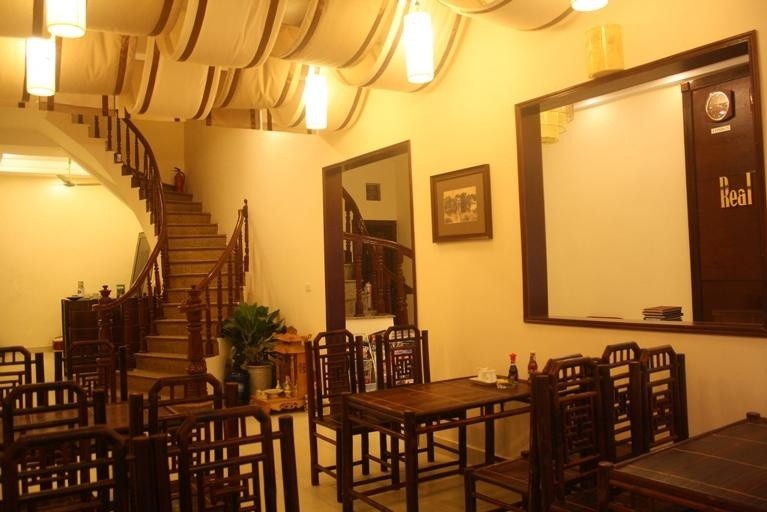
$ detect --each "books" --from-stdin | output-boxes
[642,303,683,323]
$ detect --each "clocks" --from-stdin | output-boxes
[703,91,735,123]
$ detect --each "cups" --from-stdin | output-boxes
[478,366,496,382]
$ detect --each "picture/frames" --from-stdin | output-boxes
[430,165,495,244]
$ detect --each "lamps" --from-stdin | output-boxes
[406,1,434,82]
[26,0,87,98]
[305,60,328,132]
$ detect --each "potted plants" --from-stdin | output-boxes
[218,301,286,395]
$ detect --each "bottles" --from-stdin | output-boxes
[527,351,537,384]
[77,280,84,297]
[505,351,519,383]
[116,284,125,298]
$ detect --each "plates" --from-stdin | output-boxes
[66,296,82,300]
[263,389,283,394]
[469,376,503,384]
